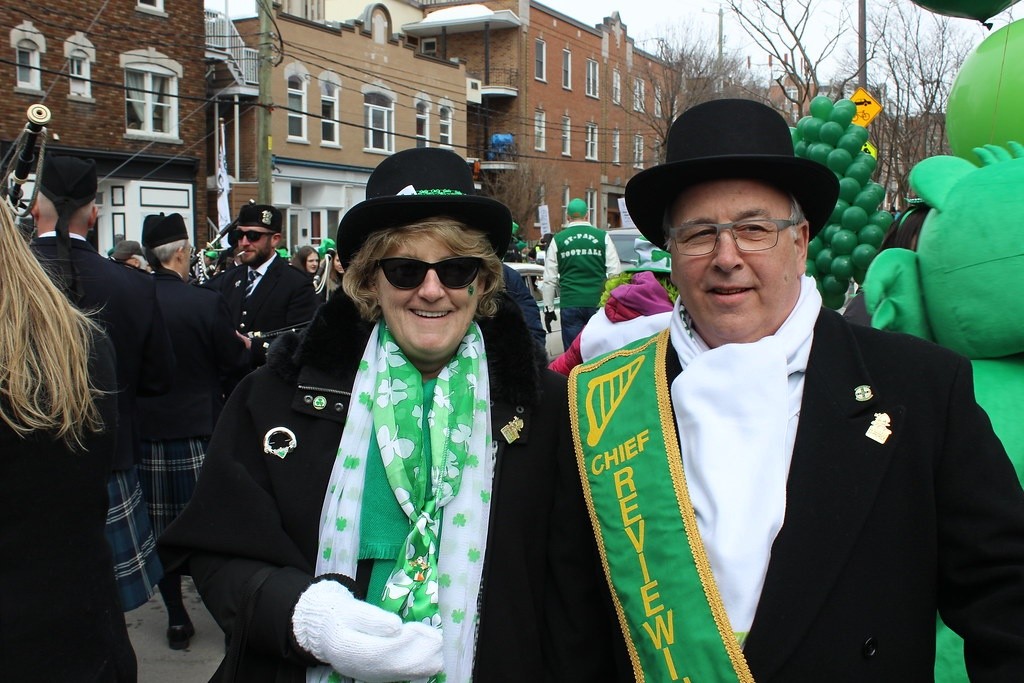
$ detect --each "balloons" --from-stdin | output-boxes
[946,18,1024,168]
[788,96,894,310]
[911,0,1021,23]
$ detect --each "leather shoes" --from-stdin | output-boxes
[167,621,195,650]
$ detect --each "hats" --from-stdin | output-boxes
[109,240,142,260]
[142,212,189,276]
[567,198,587,216]
[228,224,238,252]
[238,204,282,233]
[40,157,97,290]
[625,98,841,259]
[335,147,512,273]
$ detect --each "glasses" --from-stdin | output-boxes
[667,218,797,256]
[233,230,277,242]
[367,256,485,290]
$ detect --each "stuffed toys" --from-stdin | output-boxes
[863,141,1024,683]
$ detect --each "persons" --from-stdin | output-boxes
[290,245,320,282]
[109,241,147,270]
[204,252,218,278]
[141,212,237,650]
[0,194,139,683]
[312,248,345,303]
[204,204,316,430]
[23,155,164,613]
[156,149,564,683]
[542,198,624,352]
[842,202,931,328]
[541,98,1024,683]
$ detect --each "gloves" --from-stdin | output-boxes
[544,306,557,333]
[291,580,443,683]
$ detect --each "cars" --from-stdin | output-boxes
[603,228,661,271]
[502,261,562,312]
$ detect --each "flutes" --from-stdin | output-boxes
[0,99,338,358]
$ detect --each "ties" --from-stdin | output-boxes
[243,271,261,302]
